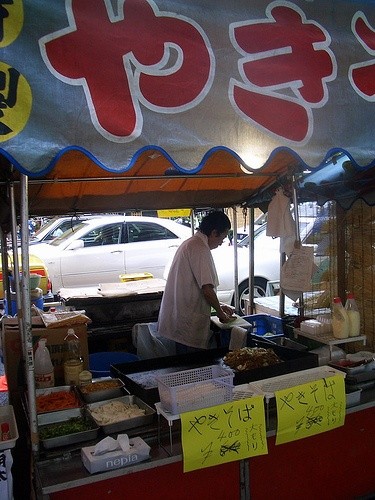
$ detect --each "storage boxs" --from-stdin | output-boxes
[240,313,284,344]
[2,316,90,405]
[155,365,235,415]
[0,405,19,451]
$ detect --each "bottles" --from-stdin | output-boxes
[331,297,349,339]
[62,329,84,386]
[345,292,360,337]
[34,341,55,388]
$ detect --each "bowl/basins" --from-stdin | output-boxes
[18,273,42,290]
[89,351,140,378]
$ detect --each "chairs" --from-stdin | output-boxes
[138,227,157,241]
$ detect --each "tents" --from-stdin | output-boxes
[1,0,375,500]
[240,152,375,355]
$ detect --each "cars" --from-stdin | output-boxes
[230,203,325,247]
[0,216,100,253]
[163,216,336,317]
[7,216,233,298]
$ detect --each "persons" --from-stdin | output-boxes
[156,211,237,356]
[94,226,113,246]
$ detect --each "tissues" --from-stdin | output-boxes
[80,434,151,475]
